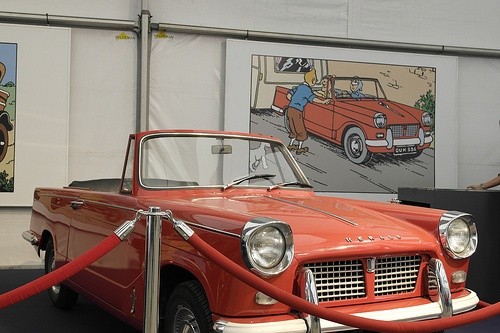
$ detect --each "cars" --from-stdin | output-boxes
[22,129,479,333]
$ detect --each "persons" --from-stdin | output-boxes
[466,173,500,190]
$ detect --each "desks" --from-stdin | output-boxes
[397,186,500,305]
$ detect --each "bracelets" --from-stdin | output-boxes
[480,183,483,188]
